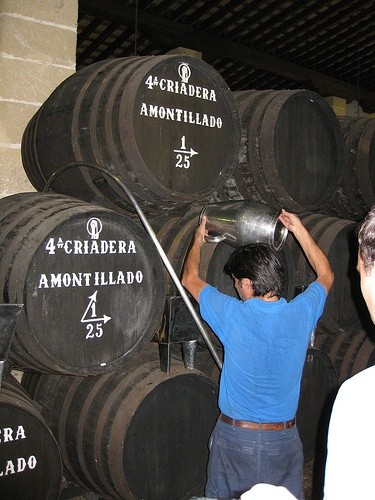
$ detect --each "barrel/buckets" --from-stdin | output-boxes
[188,88,342,213]
[316,322,375,385]
[0,193,165,377]
[0,370,64,500]
[20,55,241,223]
[194,335,339,464]
[318,111,375,220]
[281,211,370,332]
[137,213,297,348]
[22,348,221,500]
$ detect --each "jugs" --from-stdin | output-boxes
[199,200,288,252]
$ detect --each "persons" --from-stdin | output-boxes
[238,204,374,500]
[181,207,335,500]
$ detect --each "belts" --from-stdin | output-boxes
[218,413,295,431]
